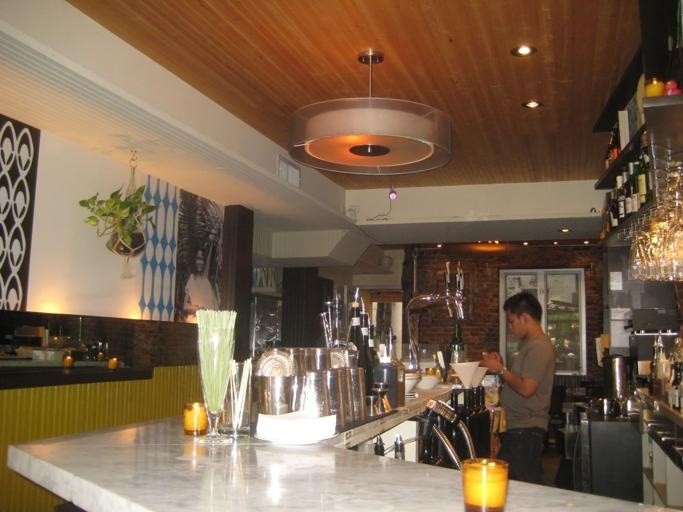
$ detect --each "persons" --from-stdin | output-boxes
[182,227,221,325]
[478,292,555,484]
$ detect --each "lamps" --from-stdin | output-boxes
[288,49,452,174]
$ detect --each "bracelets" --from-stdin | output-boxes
[496,367,506,377]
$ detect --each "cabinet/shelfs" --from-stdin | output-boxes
[593,45,655,238]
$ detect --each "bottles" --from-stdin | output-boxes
[650,336,683,413]
[346,302,397,412]
[451,322,464,364]
[598,151,651,240]
[444,385,491,468]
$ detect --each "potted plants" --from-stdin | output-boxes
[79,149,162,278]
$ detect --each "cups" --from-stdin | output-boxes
[183,400,207,435]
[259,347,367,431]
[462,457,509,512]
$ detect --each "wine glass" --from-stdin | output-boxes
[625,164,683,283]
